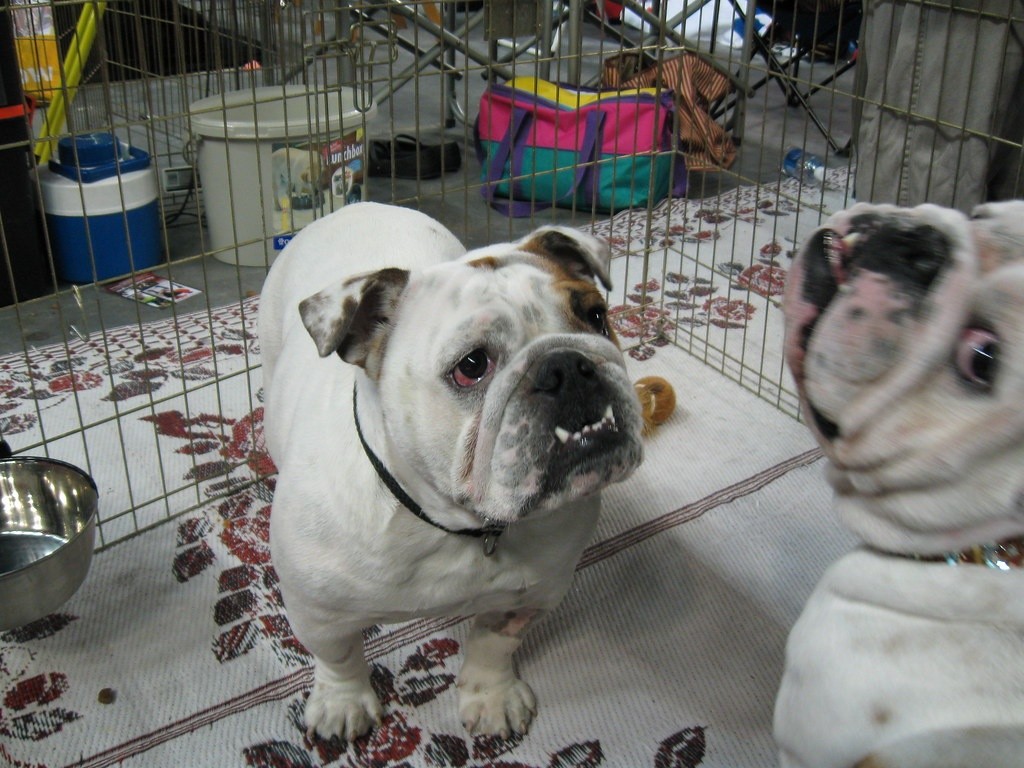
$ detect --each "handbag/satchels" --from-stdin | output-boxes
[602,50,737,172]
[479,76,688,219]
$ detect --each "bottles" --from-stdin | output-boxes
[777,143,829,187]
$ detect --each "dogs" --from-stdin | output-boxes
[771,196,1024,768]
[254,198,647,751]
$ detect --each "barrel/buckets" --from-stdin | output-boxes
[183,84,377,267]
[28,133,165,283]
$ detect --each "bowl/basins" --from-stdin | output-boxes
[1,459,99,630]
[57,134,120,168]
